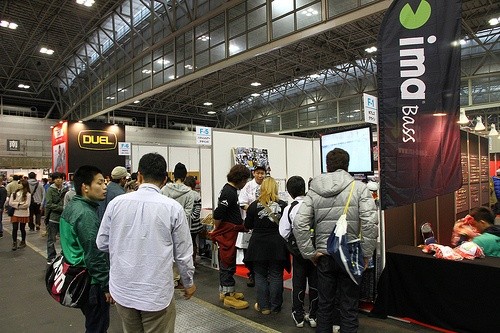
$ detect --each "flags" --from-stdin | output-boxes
[377,0,461,209]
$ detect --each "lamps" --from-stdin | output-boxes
[458,108,500,140]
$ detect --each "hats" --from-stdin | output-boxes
[110,166,127,179]
[174,162,187,179]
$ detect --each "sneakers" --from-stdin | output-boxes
[220,291,245,301]
[254,303,270,314]
[12,242,18,250]
[304,313,317,328]
[224,296,249,309]
[333,326,341,333]
[291,311,304,328]
[0,234,4,239]
[18,241,26,247]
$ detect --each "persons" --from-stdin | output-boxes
[9,179,31,251]
[293,147,379,333]
[492,168,500,213]
[59,161,119,333]
[2,172,50,236]
[43,171,69,265]
[454,206,500,257]
[200,164,317,328]
[96,153,197,333]
[0,183,8,237]
[98,162,207,291]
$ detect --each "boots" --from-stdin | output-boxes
[247,273,255,287]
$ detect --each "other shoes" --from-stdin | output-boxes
[36,224,40,231]
[46,257,57,264]
[29,228,35,231]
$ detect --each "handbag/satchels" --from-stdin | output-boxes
[45,250,91,308]
[286,232,301,258]
[327,182,365,288]
[7,192,16,216]
[29,195,41,212]
